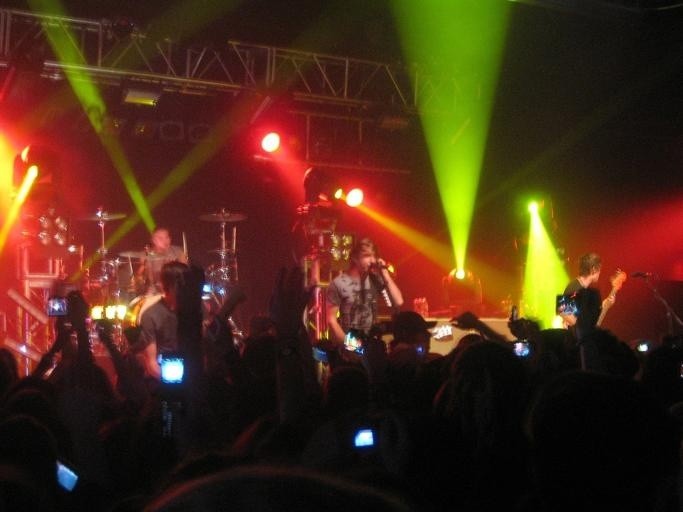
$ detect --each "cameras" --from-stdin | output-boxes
[636,342,649,352]
[555,295,579,315]
[47,296,70,316]
[512,341,531,357]
[160,351,186,391]
[345,332,369,354]
[55,457,79,493]
[353,428,375,449]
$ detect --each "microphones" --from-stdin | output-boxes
[369,264,388,269]
[630,271,649,278]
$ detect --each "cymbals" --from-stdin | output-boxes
[118,248,178,262]
[73,211,127,221]
[197,212,250,223]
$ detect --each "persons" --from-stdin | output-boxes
[1,227,682,511]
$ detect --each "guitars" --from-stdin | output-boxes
[595,266,627,327]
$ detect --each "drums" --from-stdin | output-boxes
[204,267,238,290]
[209,249,236,268]
[104,302,126,323]
[122,292,165,345]
[90,303,106,324]
[85,255,120,288]
